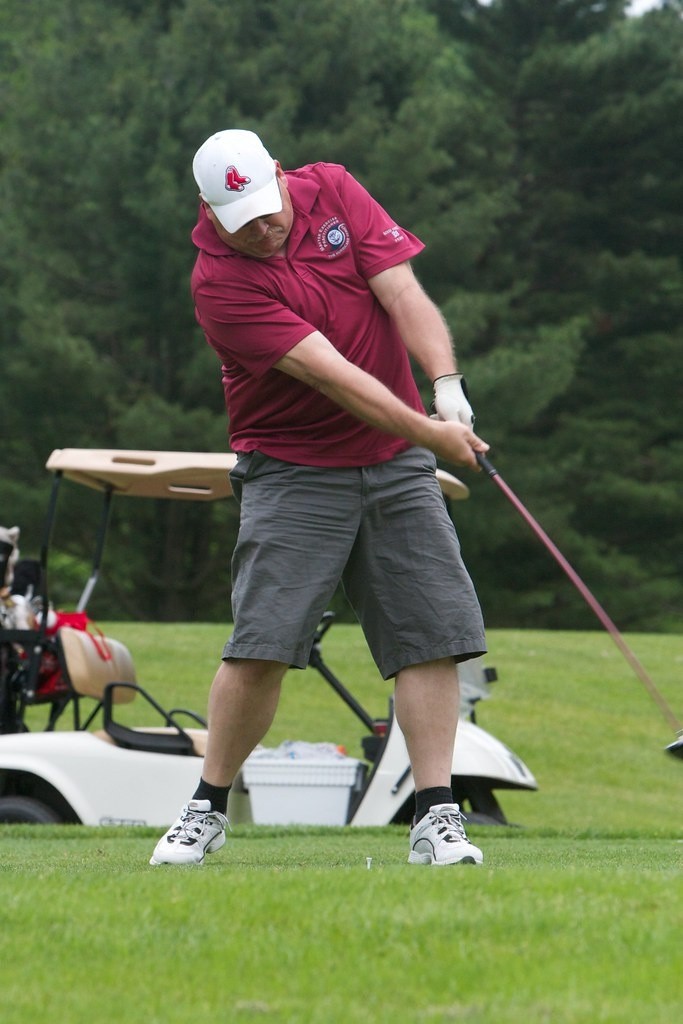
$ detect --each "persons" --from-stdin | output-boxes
[152,130,490,865]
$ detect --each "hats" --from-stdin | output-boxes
[192,128,282,234]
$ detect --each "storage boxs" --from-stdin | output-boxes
[241,756,370,827]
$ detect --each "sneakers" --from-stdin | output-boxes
[148,798,234,867]
[406,801,484,866]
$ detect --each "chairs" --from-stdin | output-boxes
[56,627,210,756]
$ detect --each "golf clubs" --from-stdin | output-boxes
[474,448,683,763]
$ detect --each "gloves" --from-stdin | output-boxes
[428,373,476,436]
